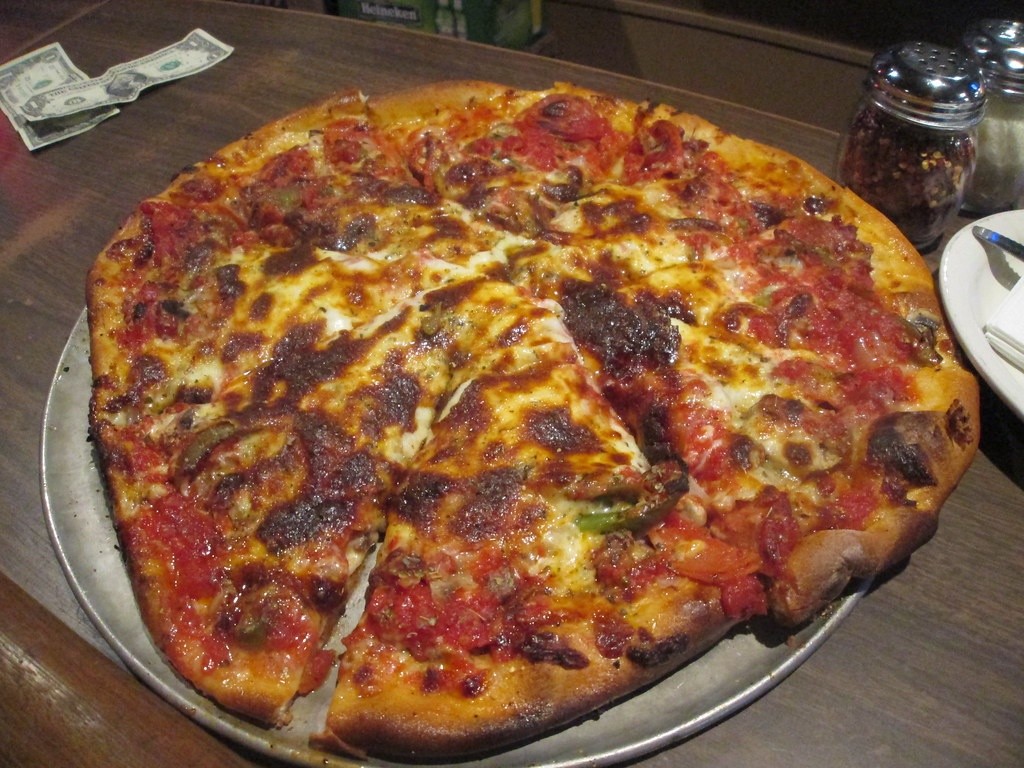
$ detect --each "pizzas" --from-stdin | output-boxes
[86,82,982,762]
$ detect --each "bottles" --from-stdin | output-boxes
[962,18,1024,219]
[833,40,989,251]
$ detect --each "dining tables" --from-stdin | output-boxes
[0,0,1024,768]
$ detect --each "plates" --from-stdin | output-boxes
[40,305,870,766]
[936,207,1024,420]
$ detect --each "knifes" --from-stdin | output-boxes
[973,226,1024,262]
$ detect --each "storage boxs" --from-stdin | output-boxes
[337,0,546,50]
